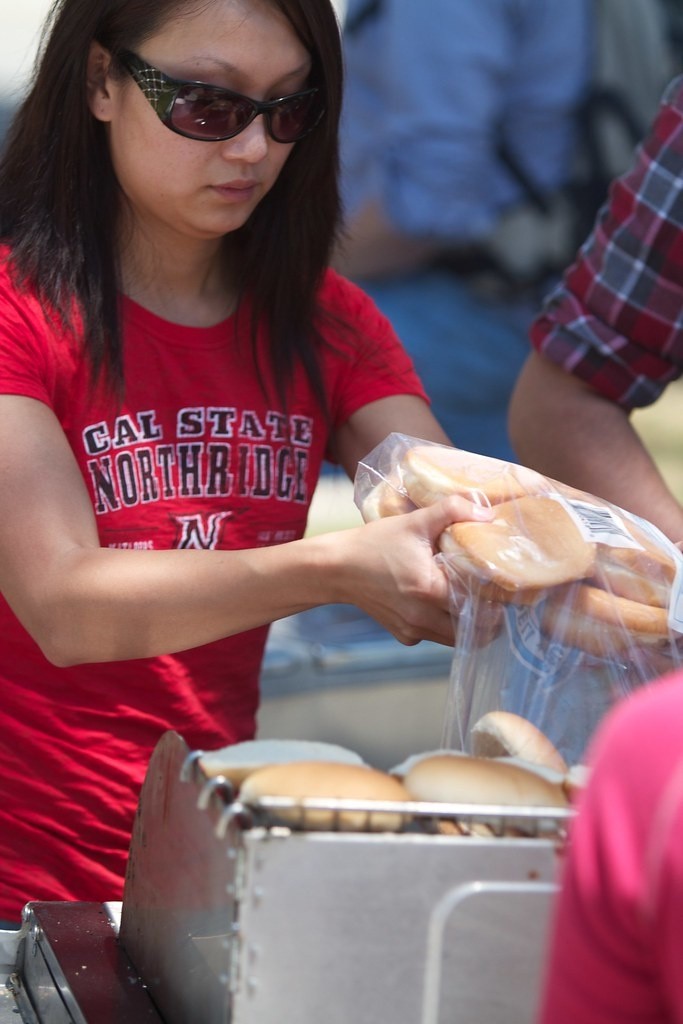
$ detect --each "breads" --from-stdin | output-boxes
[198,710,592,838]
[362,444,683,667]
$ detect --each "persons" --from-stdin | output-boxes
[535,669,683,1024]
[0,0,507,930]
[314,0,602,481]
[508,74,683,559]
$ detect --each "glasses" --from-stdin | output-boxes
[101,37,328,144]
